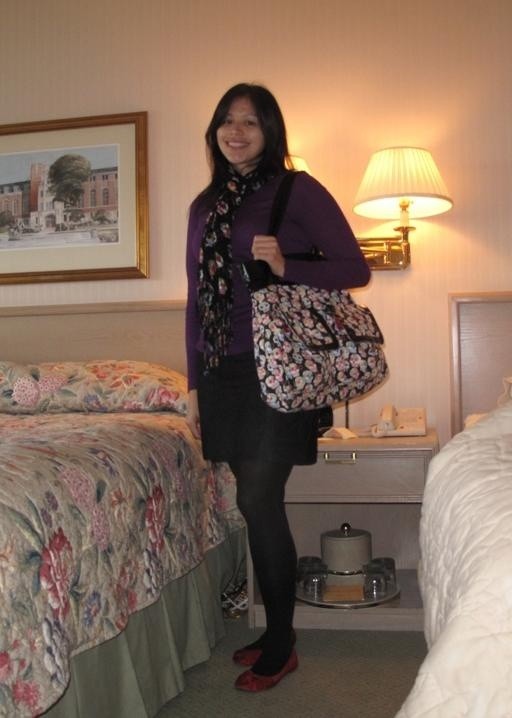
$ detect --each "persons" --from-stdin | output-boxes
[185,83,373,695]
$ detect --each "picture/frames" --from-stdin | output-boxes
[0,111,149,285]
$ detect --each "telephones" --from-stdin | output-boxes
[377,404,426,437]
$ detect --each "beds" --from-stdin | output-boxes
[1,300,247,718]
[394,290,511,718]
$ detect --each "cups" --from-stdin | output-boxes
[297,556,329,601]
[362,557,397,600]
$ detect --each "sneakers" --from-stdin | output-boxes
[222,582,249,618]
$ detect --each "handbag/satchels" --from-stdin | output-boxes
[250,284,389,414]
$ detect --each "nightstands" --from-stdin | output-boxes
[247,428,440,630]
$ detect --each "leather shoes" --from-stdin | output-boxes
[233,628,296,666]
[236,648,297,690]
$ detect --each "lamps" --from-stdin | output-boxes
[284,146,454,271]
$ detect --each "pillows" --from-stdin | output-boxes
[0,357,188,415]
